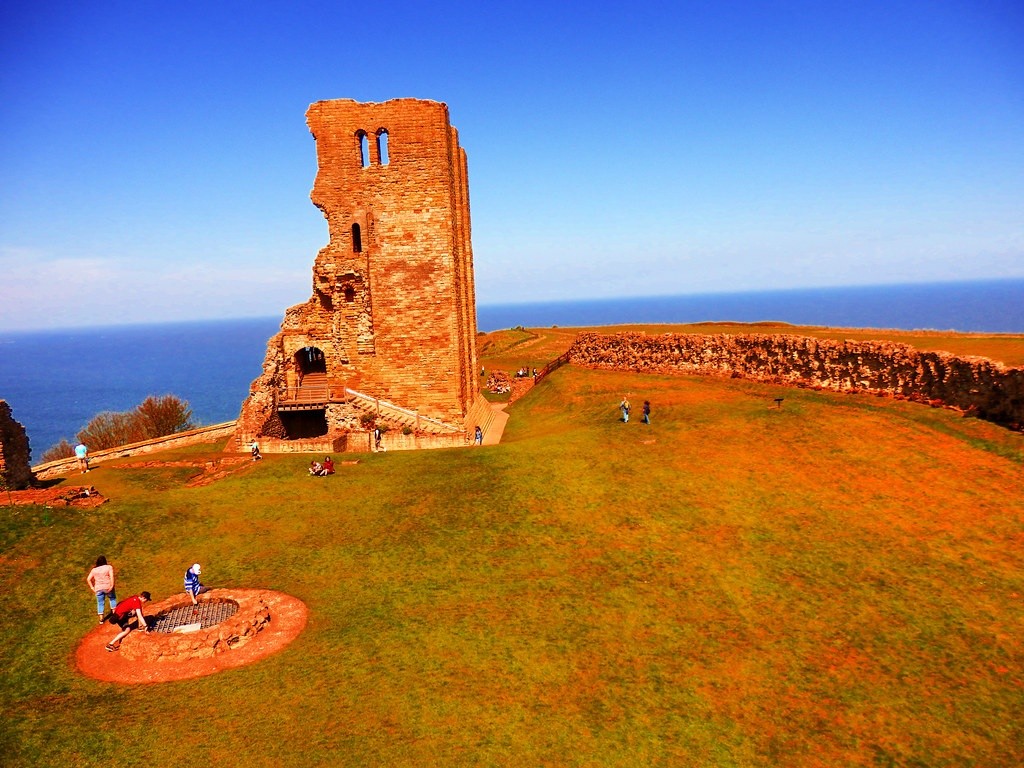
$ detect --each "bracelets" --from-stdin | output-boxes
[91,587,94,590]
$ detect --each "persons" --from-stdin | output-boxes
[480,363,485,377]
[619,396,632,424]
[514,365,537,378]
[86,555,117,625]
[74,442,91,475]
[642,399,652,425]
[183,563,206,606]
[374,428,386,453]
[105,591,152,652]
[251,438,262,462]
[308,455,335,477]
[473,425,483,447]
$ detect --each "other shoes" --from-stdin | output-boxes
[99,618,105,625]
[374,450,379,453]
[80,470,84,474]
[86,469,91,473]
[383,447,387,453]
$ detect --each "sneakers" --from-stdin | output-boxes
[104,644,118,653]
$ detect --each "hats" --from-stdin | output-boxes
[141,591,151,601]
[192,563,202,575]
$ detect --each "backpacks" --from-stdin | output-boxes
[624,402,629,410]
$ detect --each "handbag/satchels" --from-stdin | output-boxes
[108,615,117,625]
[252,446,259,456]
